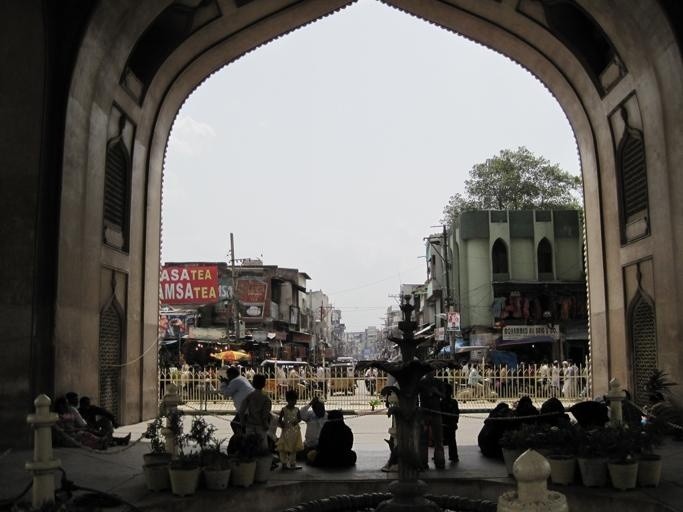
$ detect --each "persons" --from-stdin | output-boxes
[246,365,255,384]
[364,366,377,383]
[462,361,580,399]
[216,366,357,473]
[277,362,330,400]
[50,392,132,451]
[377,353,459,472]
[170,360,202,388]
[477,389,678,461]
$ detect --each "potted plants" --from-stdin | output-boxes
[143,408,274,494]
[494,423,666,491]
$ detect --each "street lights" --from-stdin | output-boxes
[170,318,182,368]
[429,225,451,312]
[543,310,560,360]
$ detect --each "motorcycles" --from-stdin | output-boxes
[366,376,376,395]
[456,377,499,403]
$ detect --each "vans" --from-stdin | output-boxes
[215,357,358,400]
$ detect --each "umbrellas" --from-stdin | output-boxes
[209,349,250,363]
